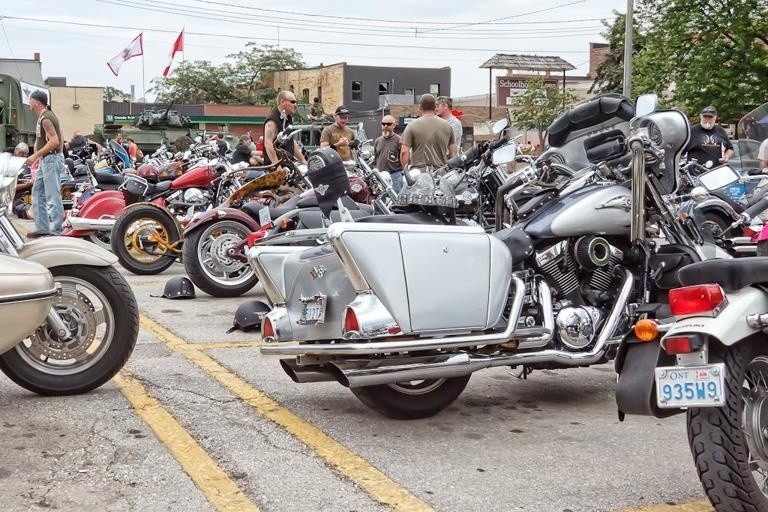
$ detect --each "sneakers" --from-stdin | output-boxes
[26,231,46,238]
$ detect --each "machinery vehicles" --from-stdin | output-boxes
[93,97,209,155]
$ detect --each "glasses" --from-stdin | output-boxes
[285,99,296,104]
[382,123,393,126]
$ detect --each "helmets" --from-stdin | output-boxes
[164,276,194,299]
[137,164,159,184]
[233,300,271,329]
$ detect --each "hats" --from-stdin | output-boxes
[336,106,351,117]
[701,107,717,118]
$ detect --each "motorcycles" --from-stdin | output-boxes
[242,88,740,423]
[0,148,139,398]
[606,191,766,415]
[651,101,767,512]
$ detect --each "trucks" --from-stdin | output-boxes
[0,72,51,157]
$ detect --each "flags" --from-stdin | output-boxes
[162,32,183,76]
[106,32,143,77]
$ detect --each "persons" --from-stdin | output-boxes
[201,89,463,196]
[63,129,139,169]
[756,138,768,181]
[527,140,532,156]
[516,141,524,155]
[24,91,65,238]
[13,142,33,214]
[679,106,735,181]
